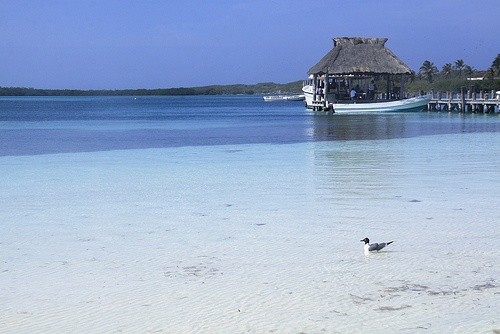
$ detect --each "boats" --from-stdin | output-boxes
[332,93,433,112]
[262,94,305,102]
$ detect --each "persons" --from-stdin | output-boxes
[370,80,377,97]
[421,89,426,95]
[317,86,324,98]
[350,88,357,103]
[329,79,336,89]
[356,85,362,93]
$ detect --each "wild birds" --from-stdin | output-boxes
[360,237,394,257]
